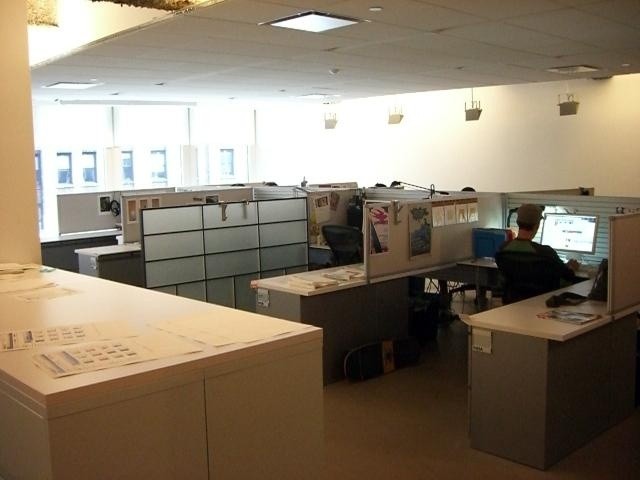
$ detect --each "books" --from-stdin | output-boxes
[536,310,601,325]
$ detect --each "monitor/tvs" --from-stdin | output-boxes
[539,212,599,271]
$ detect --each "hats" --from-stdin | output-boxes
[517,204,544,224]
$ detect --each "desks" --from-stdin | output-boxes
[458,277,640,472]
[248,260,459,390]
[74,244,144,288]
[456,257,593,317]
[39,228,122,275]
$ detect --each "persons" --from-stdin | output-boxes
[499,204,579,294]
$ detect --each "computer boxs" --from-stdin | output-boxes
[347,206,363,231]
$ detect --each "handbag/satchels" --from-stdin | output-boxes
[588,261,607,300]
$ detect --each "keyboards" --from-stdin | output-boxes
[561,257,592,267]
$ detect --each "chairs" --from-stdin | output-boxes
[320,223,363,266]
[495,249,561,308]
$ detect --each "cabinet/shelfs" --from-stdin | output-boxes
[1,259,326,480]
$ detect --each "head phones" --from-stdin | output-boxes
[579,187,589,196]
